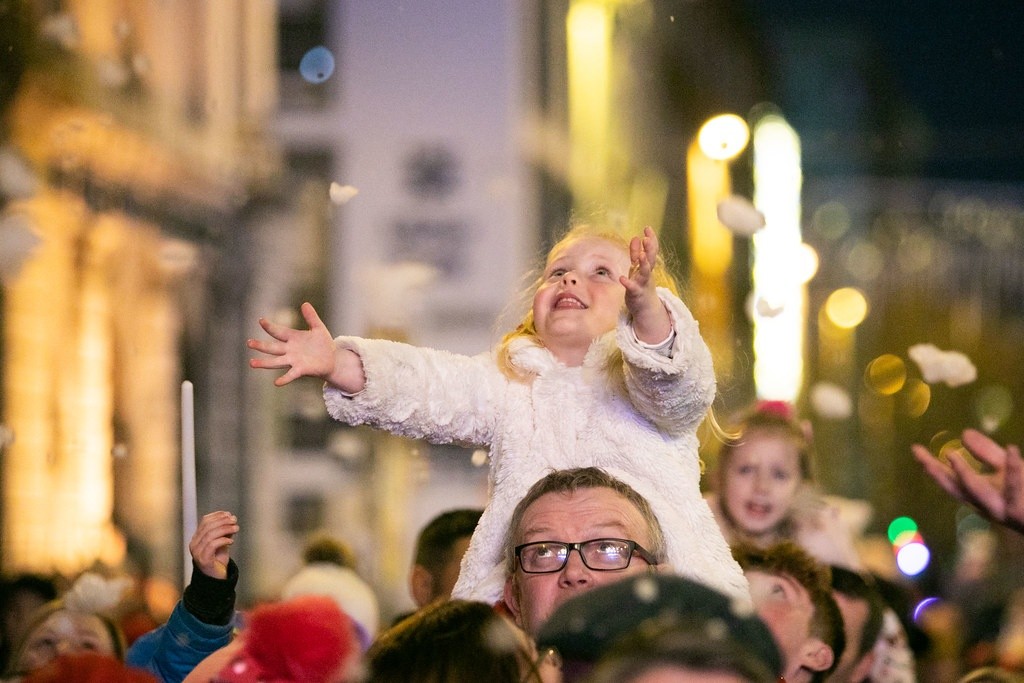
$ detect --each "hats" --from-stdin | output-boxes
[281,562,380,656]
[180,594,365,683]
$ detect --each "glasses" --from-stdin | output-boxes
[514,538,653,573]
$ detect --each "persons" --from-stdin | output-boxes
[698,399,867,576]
[1,467,1021,683]
[909,425,1024,530]
[246,224,754,623]
